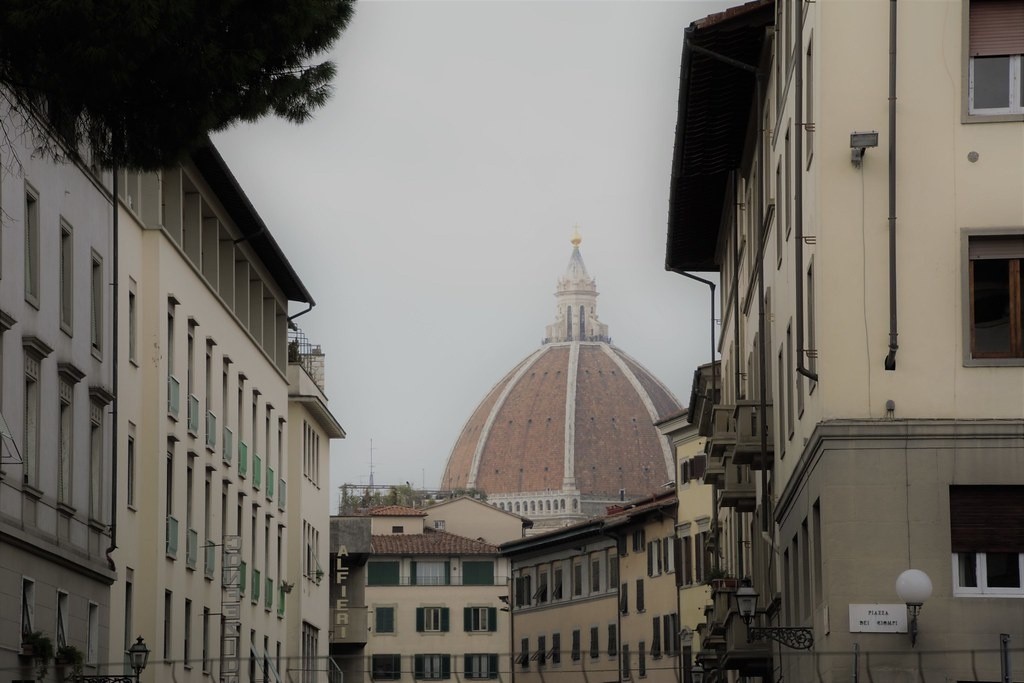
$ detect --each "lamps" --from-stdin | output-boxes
[65,635,151,682]
[850,132,878,164]
[735,576,815,653]
[897,569,933,648]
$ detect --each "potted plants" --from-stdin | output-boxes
[57,645,86,683]
[701,567,729,590]
[22,630,55,683]
